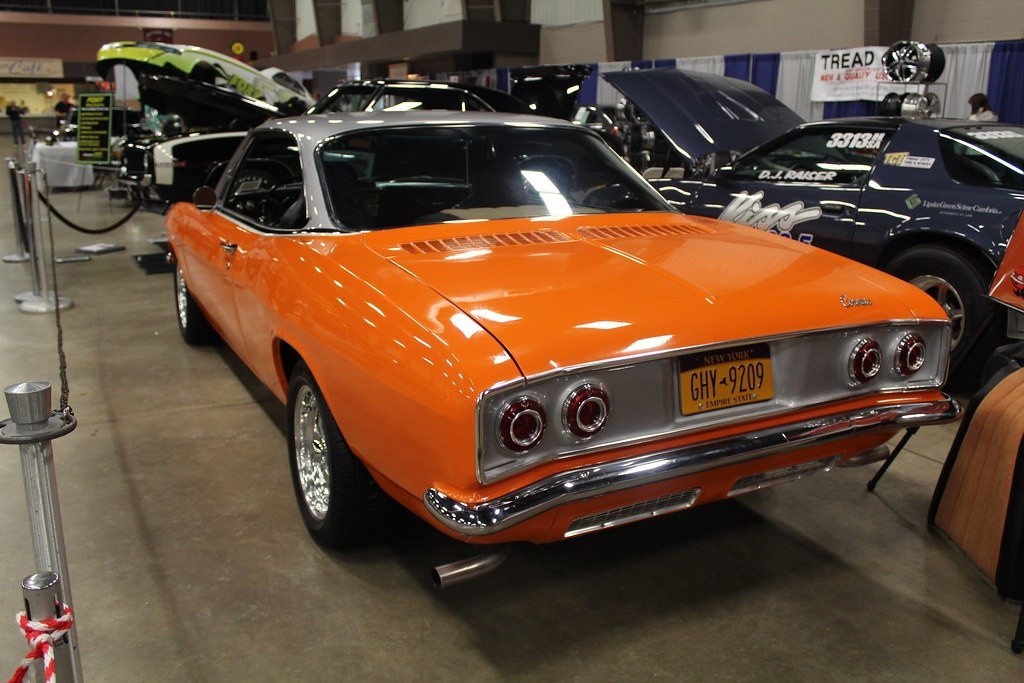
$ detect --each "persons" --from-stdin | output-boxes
[6,101,27,146]
[968,93,1000,123]
[55,93,73,129]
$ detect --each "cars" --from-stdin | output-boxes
[573,104,652,148]
[96,41,574,204]
[59,108,143,143]
[150,108,964,588]
[599,68,1023,377]
